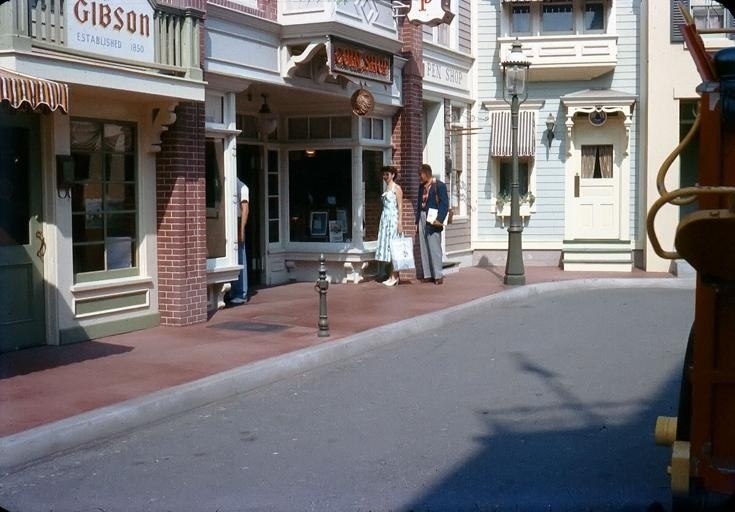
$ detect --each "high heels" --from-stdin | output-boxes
[382,277,399,286]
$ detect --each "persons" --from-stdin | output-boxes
[414,164,450,285]
[215,174,252,305]
[374,165,405,287]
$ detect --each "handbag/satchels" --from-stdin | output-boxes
[389,231,415,272]
[447,208,453,224]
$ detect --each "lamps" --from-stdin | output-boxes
[257,94,277,137]
[546,112,556,147]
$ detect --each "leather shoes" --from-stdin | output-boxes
[421,277,443,285]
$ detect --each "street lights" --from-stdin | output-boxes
[499,35,532,286]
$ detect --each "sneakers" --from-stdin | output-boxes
[229,296,247,304]
